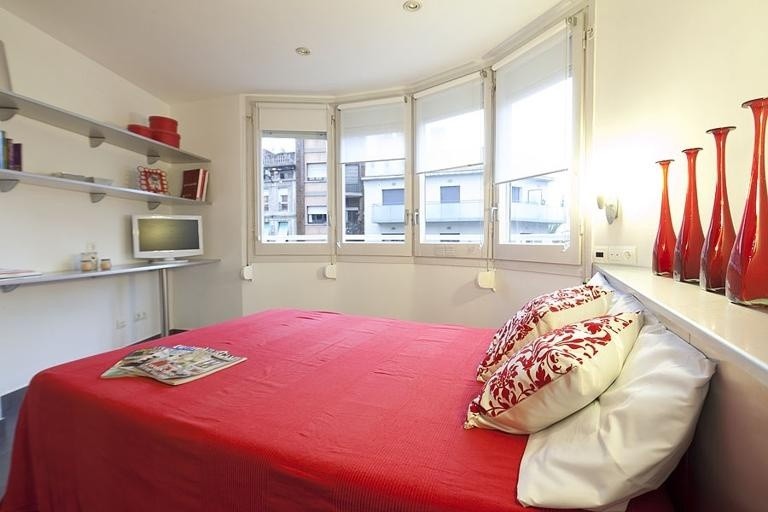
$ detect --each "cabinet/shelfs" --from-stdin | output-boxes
[0,89,218,292]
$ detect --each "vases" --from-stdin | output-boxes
[675,145,708,280]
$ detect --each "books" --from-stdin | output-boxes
[0,130,22,171]
[181,168,210,201]
[101,343,247,387]
[0,270,42,279]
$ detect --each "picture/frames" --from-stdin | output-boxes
[136,164,168,194]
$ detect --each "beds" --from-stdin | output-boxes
[1,260,768,512]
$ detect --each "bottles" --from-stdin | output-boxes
[673,147,706,283]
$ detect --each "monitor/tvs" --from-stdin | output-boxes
[132,214,204,265]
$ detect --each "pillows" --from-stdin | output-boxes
[458,308,646,437]
[475,282,615,381]
[515,320,720,510]
[585,271,624,314]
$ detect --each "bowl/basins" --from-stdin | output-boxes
[127,115,181,149]
